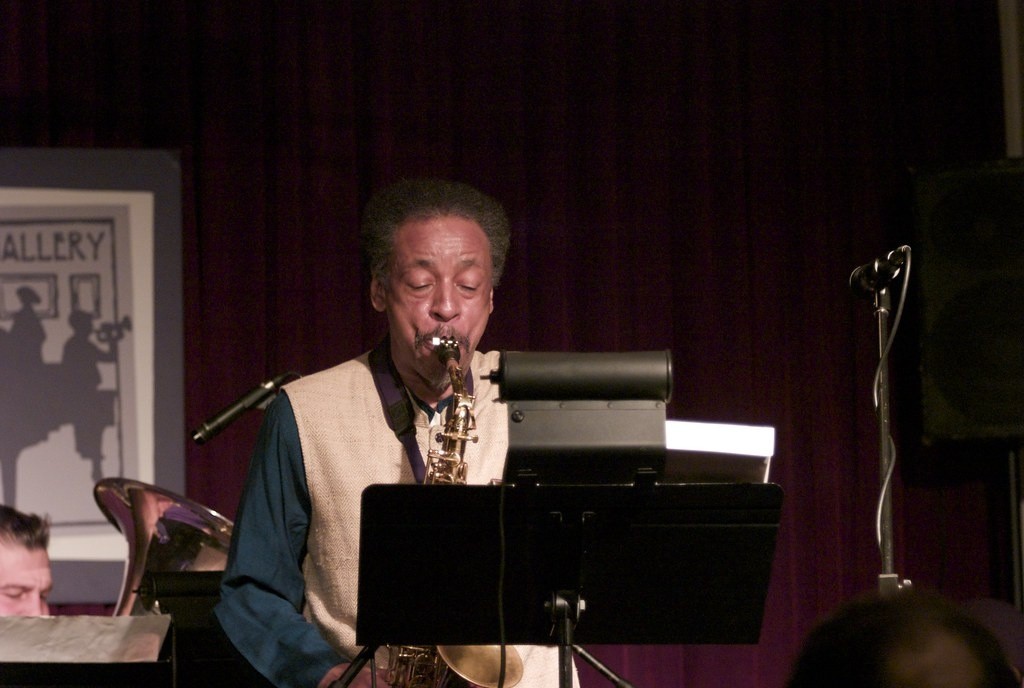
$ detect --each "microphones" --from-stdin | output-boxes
[191,373,289,446]
[849,245,911,299]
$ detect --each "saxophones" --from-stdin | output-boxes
[380,335,527,688]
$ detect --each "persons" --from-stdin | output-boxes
[0,505,53,614]
[787,602,1024,688]
[214,178,581,688]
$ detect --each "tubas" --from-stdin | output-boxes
[90,476,238,620]
